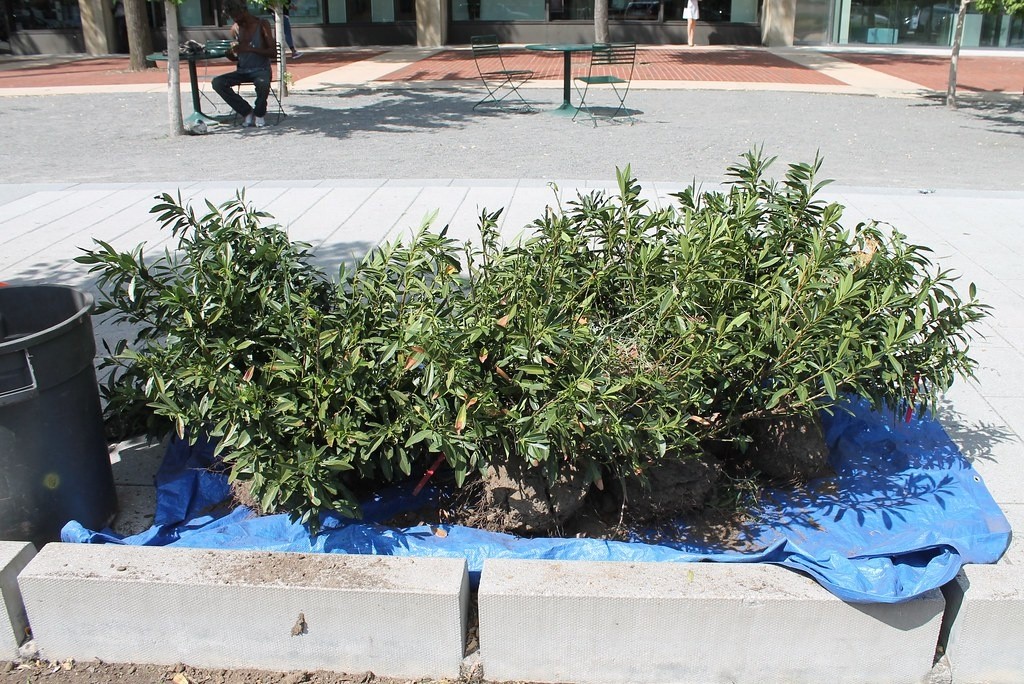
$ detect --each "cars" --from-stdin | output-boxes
[850,3,888,31]
[910,4,958,34]
[624,0,723,22]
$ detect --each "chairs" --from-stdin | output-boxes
[230,41,286,126]
[571,41,637,129]
[470,34,538,111]
[196,39,238,112]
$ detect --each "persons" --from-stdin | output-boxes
[267,0,304,59]
[683,0,703,47]
[211,0,276,127]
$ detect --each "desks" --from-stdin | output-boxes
[145,51,229,128]
[525,43,609,118]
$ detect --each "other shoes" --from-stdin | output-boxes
[255,116,265,127]
[242,112,253,127]
[292,52,303,59]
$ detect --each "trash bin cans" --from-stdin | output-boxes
[0,279,123,555]
